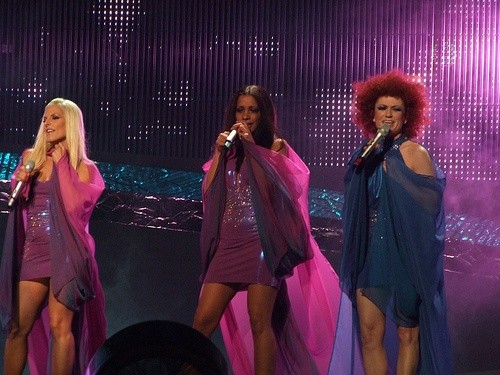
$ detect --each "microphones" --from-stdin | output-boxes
[8,160,35,206]
[223,129,238,149]
[358,124,390,161]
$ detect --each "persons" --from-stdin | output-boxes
[343,67,442,375]
[3,98,93,375]
[191,85,310,375]
